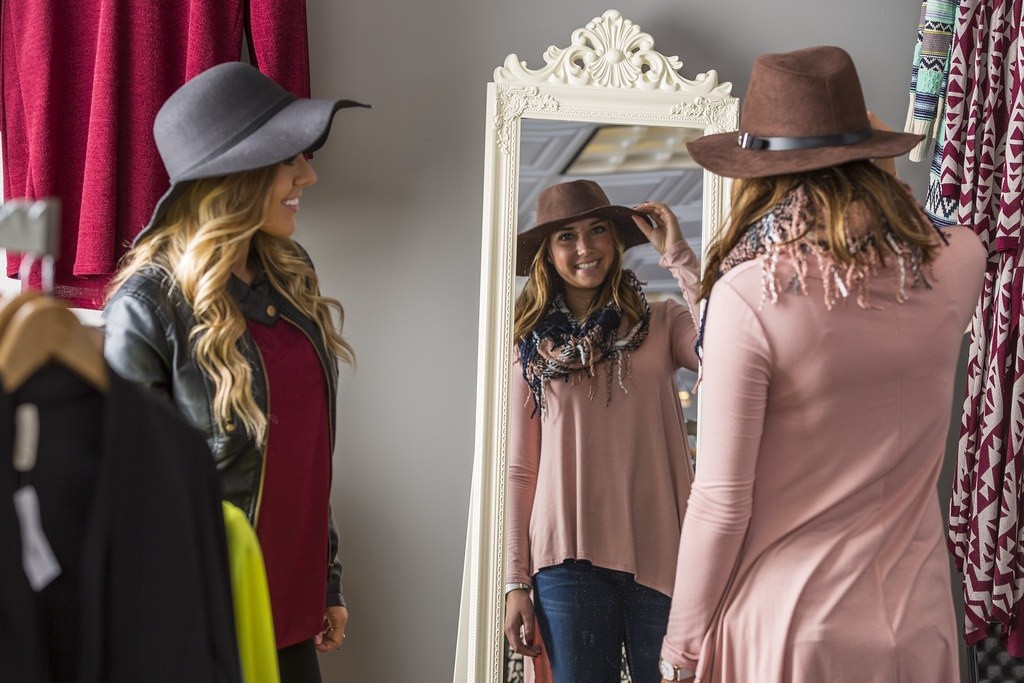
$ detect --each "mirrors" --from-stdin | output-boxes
[454,10,738,683]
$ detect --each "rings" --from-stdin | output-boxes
[329,627,334,631]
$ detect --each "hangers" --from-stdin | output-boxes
[0,198,208,475]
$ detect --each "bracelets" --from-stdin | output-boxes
[505,583,530,595]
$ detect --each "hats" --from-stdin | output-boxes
[131,61,373,248]
[686,45,926,179]
[517,181,654,278]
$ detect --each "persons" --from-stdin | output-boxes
[102,62,374,683]
[505,180,700,683]
[658,44,985,683]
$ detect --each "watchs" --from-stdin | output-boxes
[659,659,695,681]
